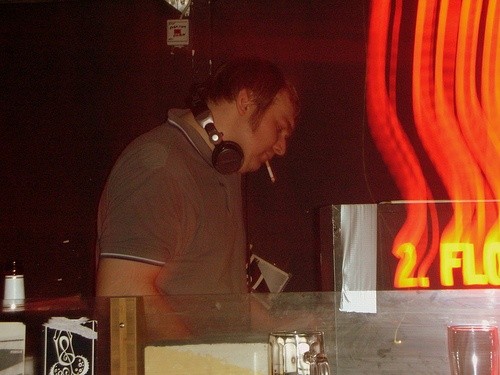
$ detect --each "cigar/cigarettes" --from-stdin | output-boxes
[266,159,276,183]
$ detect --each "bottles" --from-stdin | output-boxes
[3,261,25,308]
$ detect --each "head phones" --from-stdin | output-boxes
[184,95,244,175]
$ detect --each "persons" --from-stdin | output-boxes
[93,55,323,342]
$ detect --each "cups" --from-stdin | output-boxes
[447,326,500,375]
[269,332,329,375]
